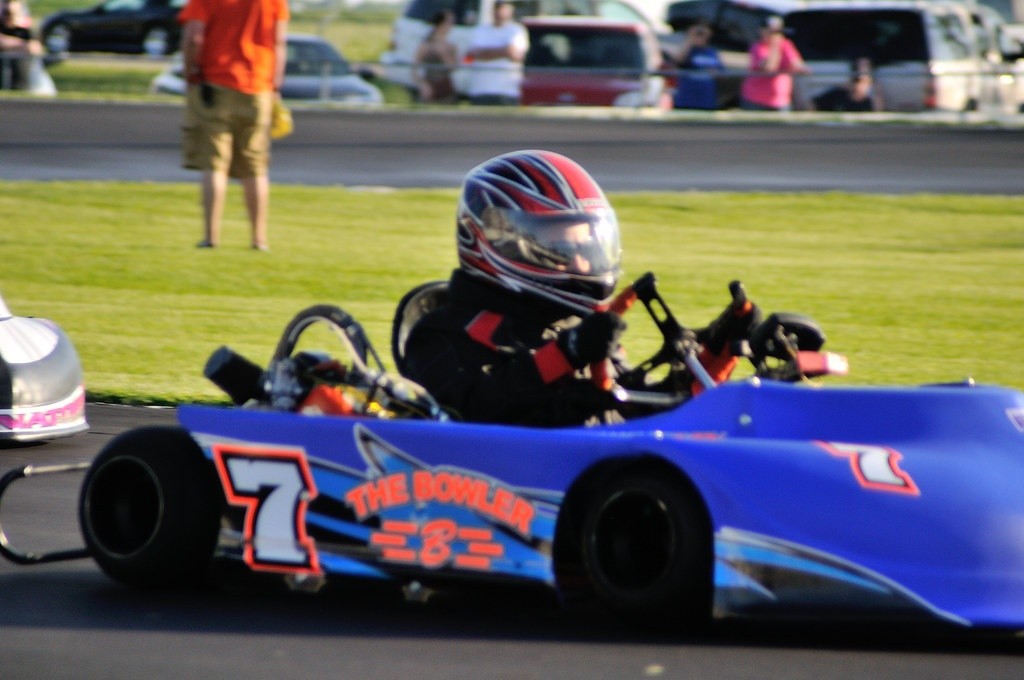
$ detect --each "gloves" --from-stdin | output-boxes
[557,312,626,371]
[704,300,761,355]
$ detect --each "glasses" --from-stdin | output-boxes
[544,242,596,263]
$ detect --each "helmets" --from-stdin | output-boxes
[456,150,620,317]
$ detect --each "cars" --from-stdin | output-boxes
[660,0,1024,114]
[37,0,188,56]
[152,35,384,104]
[524,17,674,108]
[379,1,681,106]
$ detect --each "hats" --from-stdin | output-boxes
[760,15,793,33]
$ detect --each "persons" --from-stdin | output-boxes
[671,25,726,110]
[0,0,57,96]
[178,0,291,253]
[401,150,761,429]
[807,70,881,111]
[737,15,805,112]
[459,0,529,105]
[411,9,458,104]
